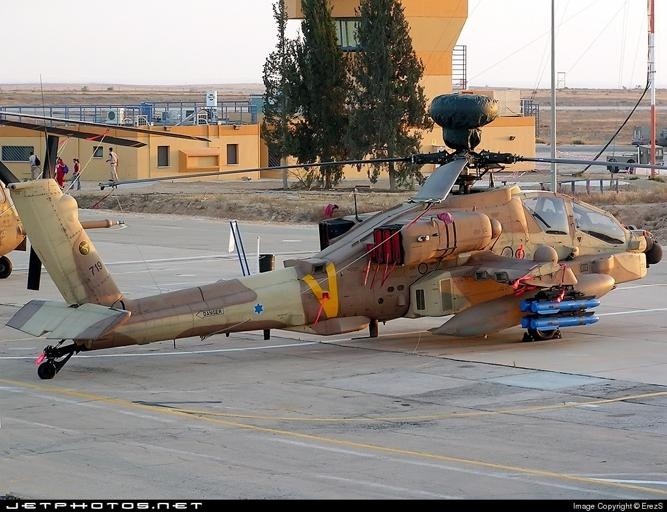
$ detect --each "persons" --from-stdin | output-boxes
[70,158,82,190]
[55,156,69,190]
[29,151,41,180]
[106,147,120,181]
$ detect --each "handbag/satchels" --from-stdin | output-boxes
[62,164,69,173]
[34,155,42,166]
